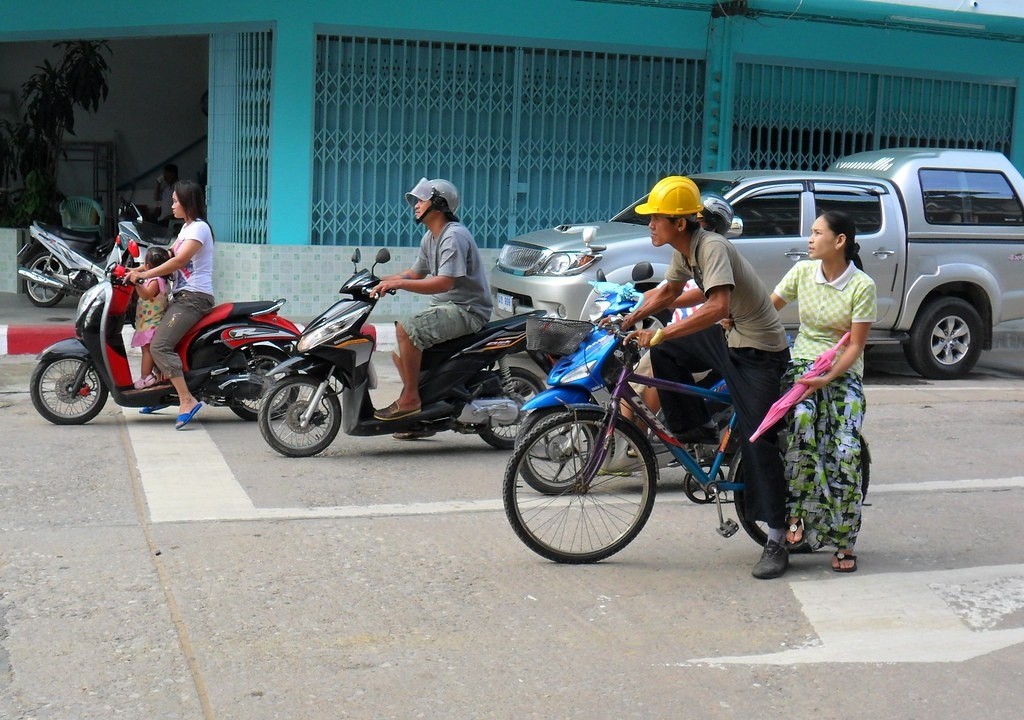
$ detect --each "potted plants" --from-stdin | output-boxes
[1,40,116,295]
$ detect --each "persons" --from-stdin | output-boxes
[596,175,791,580]
[595,190,734,477]
[720,210,877,573]
[129,180,214,430]
[369,177,493,421]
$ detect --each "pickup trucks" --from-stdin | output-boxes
[490,147,1024,380]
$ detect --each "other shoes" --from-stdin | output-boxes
[392,432,437,440]
[134,374,155,389]
[374,402,421,420]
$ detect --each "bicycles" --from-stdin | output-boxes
[501,313,871,564]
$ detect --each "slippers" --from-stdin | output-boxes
[831,552,857,572]
[175,402,202,429]
[627,449,637,456]
[139,404,169,413]
[786,518,805,549]
[597,469,633,476]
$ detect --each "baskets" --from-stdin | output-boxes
[525,316,595,355]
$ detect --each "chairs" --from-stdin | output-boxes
[59,196,106,245]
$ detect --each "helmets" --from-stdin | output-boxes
[634,176,704,217]
[699,191,735,236]
[420,179,460,214]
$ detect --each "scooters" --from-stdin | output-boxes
[257,247,557,458]
[17,195,149,308]
[514,269,792,497]
[28,233,302,426]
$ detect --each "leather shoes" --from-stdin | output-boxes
[752,535,788,579]
[652,423,720,445]
